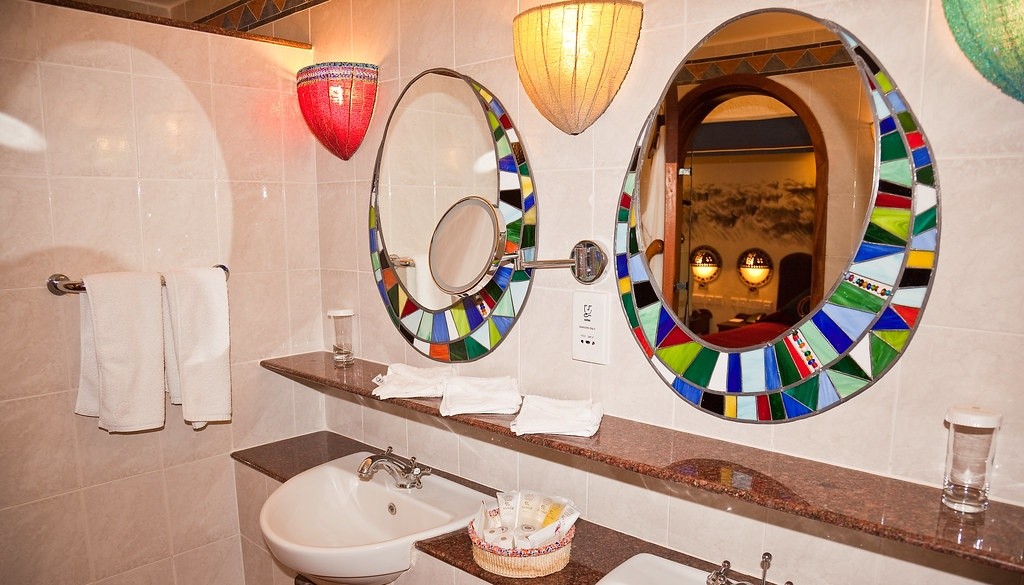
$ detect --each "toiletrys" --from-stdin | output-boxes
[474,489,584,549]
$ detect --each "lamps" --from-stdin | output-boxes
[691,245,717,285]
[740,248,771,288]
[296,62,379,161]
[941,0,1024,103]
[515,0,643,136]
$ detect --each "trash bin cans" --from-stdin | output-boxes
[717,313,750,333]
[689,308,712,335]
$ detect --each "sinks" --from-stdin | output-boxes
[259,464,453,585]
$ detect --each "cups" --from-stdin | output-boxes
[942,407,1003,513]
[327,307,356,368]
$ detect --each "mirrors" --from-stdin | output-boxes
[428,199,608,297]
[370,68,541,362]
[615,11,941,423]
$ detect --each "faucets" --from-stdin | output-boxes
[357,454,417,488]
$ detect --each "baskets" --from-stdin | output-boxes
[467,508,575,578]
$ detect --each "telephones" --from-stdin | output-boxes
[746,313,766,324]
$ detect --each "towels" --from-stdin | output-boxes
[371,363,448,400]
[439,376,522,418]
[74,267,232,435]
[509,394,605,437]
